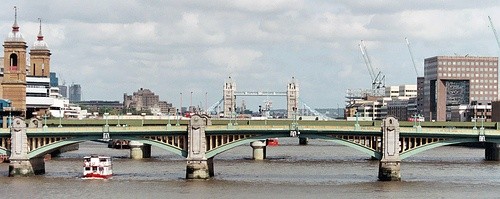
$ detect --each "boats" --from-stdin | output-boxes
[271,138,280,146]
[79,154,112,179]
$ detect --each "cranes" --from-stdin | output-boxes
[357,39,386,88]
[487,15,500,51]
[404,37,420,77]
